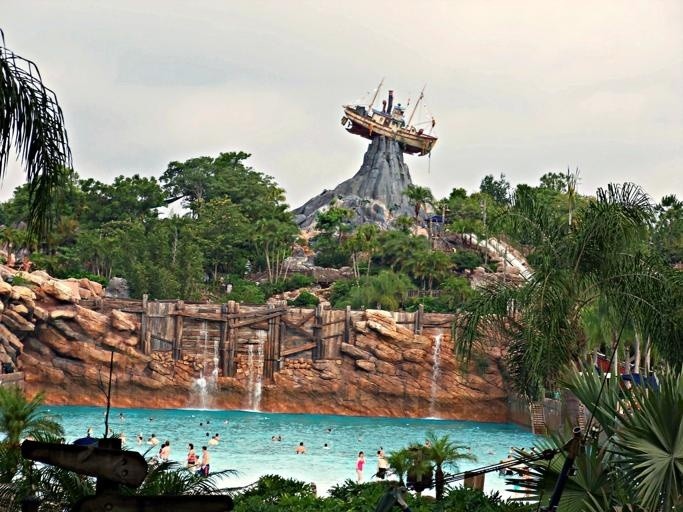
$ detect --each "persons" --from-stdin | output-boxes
[277,435,282,441]
[18,256,36,273]
[380,446,385,457]
[85,411,220,478]
[295,442,304,454]
[327,428,331,432]
[487,446,537,498]
[325,443,327,447]
[356,450,366,481]
[377,450,388,480]
[272,436,276,440]
[424,440,430,448]
